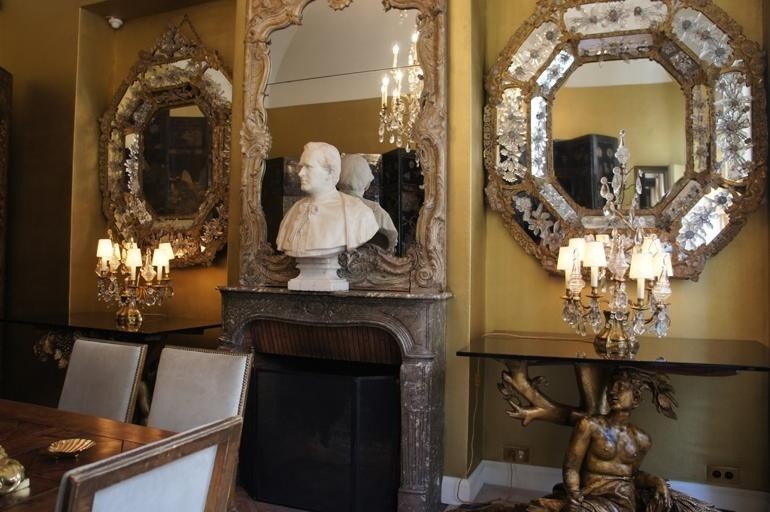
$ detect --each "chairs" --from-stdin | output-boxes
[53,337,254,512]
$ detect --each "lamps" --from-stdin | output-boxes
[378,30,424,153]
[94,133,175,333]
[556,129,673,357]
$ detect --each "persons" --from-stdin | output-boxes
[275,140,380,258]
[334,153,398,251]
[561,367,671,512]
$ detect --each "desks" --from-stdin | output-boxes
[455,329,770,512]
[8,308,225,426]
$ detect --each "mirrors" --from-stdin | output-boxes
[98,31,234,269]
[239,0,450,296]
[482,2,767,284]
